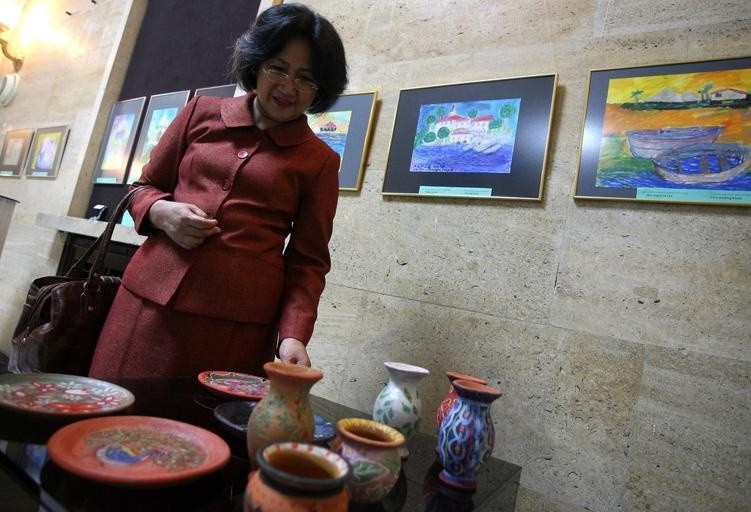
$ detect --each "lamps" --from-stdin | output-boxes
[0,0,29,75]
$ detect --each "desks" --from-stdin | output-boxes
[0,366,524,512]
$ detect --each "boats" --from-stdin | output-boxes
[626,125,727,159]
[653,144,751,185]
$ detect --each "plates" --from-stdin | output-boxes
[211,401,337,445]
[197,369,275,402]
[0,372,136,419]
[47,415,233,486]
[192,392,219,411]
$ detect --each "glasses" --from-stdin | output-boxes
[259,63,321,95]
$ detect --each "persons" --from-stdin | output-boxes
[85,3,352,387]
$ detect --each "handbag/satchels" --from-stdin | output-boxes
[6,185,155,377]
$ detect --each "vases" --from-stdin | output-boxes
[241,441,353,512]
[433,380,506,492]
[372,359,431,461]
[434,368,487,459]
[245,360,324,463]
[330,417,407,504]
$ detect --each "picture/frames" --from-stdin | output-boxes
[124,88,193,186]
[194,82,242,98]
[303,89,382,193]
[88,94,148,187]
[573,57,751,209]
[24,124,72,180]
[377,71,560,205]
[0,127,36,180]
